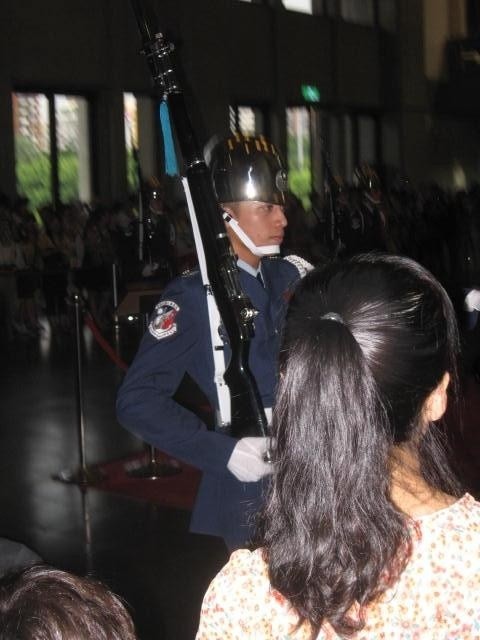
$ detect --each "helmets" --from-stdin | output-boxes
[207,133,292,209]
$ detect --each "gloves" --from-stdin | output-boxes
[225,434,280,484]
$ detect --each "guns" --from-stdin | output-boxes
[129,0,272,467]
